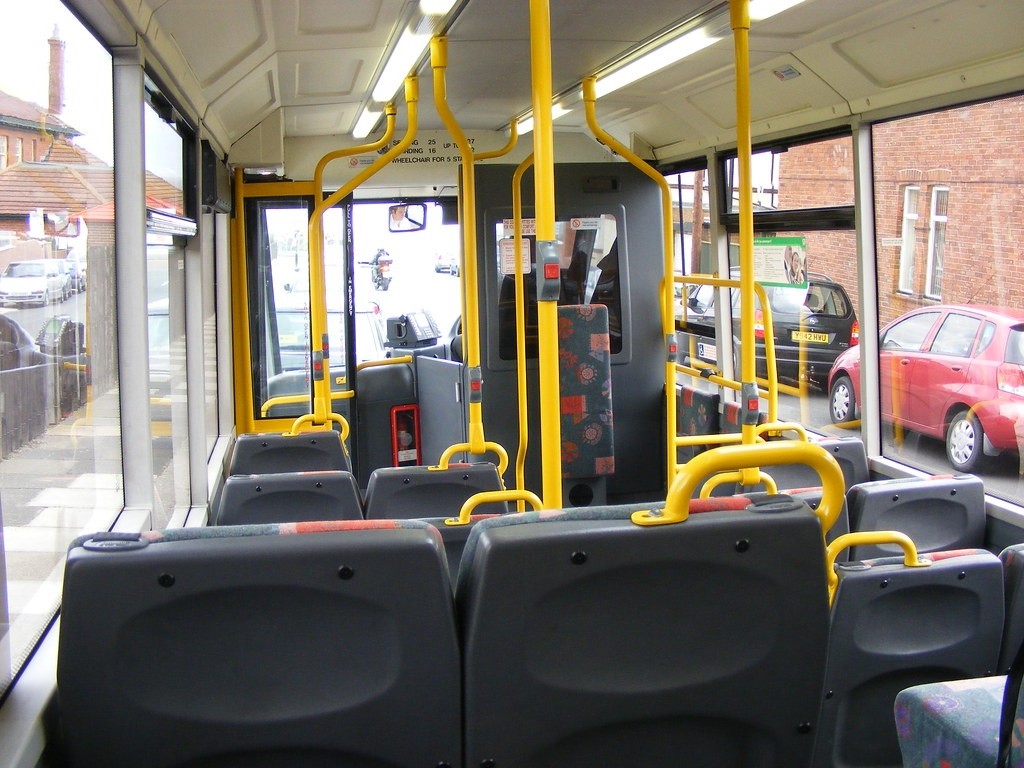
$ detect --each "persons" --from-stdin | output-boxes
[368,248,389,280]
[390,206,411,230]
[785,246,804,284]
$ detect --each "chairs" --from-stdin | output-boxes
[58,294,1024,768]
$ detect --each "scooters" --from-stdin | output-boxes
[369,255,394,291]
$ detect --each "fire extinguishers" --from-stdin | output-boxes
[397,411,417,466]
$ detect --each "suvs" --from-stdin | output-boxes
[678,266,861,396]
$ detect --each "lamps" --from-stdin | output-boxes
[351,0,806,140]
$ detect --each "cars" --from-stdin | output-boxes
[824,303,1024,475]
[266,296,391,372]
[434,249,459,276]
[0,257,88,309]
